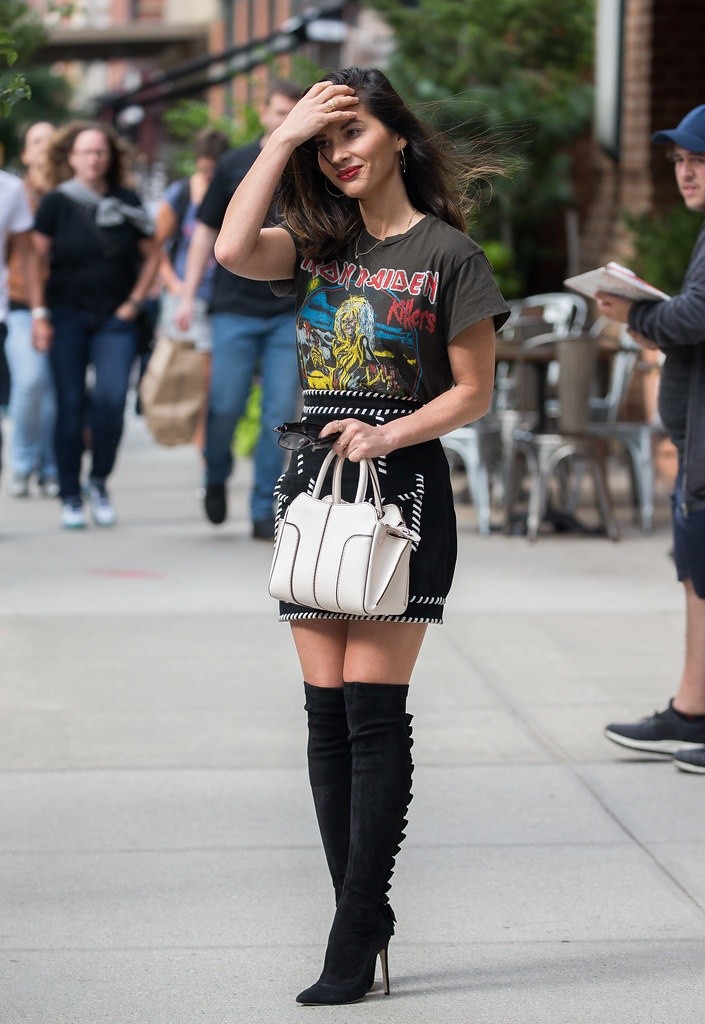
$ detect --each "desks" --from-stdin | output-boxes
[492,341,619,433]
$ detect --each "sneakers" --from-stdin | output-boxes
[672,746,705,774]
[604,698,705,754]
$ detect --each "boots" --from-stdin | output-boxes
[302,680,352,908]
[295,681,415,1007]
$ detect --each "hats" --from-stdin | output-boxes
[652,104,705,154]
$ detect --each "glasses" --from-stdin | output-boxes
[272,422,344,452]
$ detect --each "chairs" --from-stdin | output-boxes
[440,290,670,542]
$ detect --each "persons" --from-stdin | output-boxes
[598,103,704,774]
[211,68,512,1009]
[0,78,326,546]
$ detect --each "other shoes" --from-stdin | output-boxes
[203,480,226,524]
[253,528,275,541]
[88,481,116,526]
[11,474,30,495]
[62,496,86,529]
[40,480,61,496]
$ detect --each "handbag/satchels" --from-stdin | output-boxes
[268,446,422,615]
[140,322,211,446]
